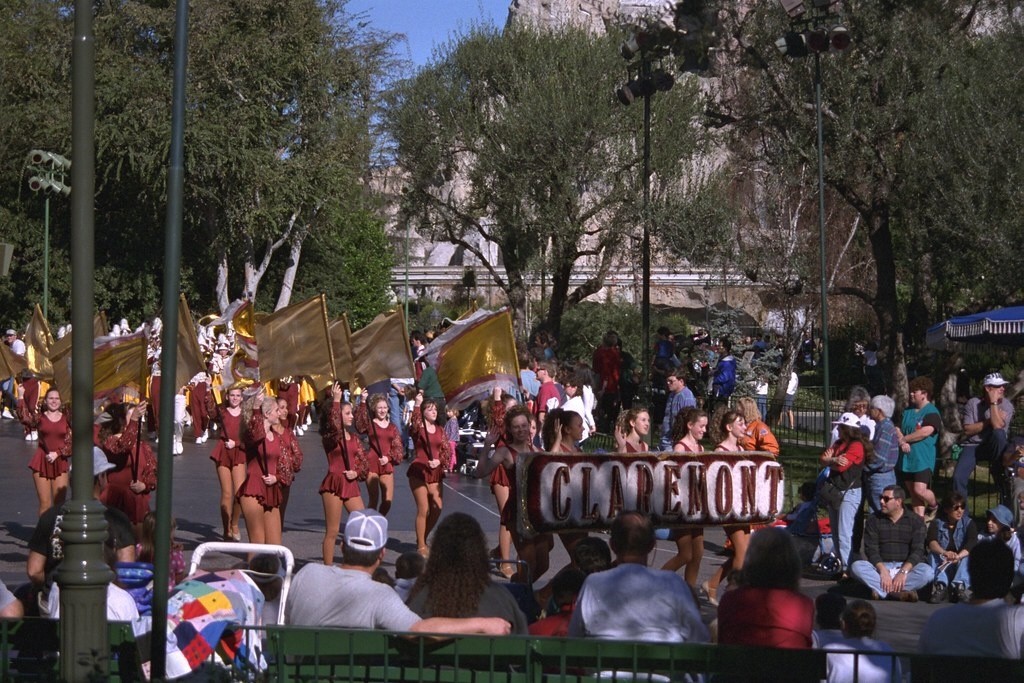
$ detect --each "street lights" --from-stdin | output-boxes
[770,0,851,451]
[616,20,675,393]
[25,149,73,320]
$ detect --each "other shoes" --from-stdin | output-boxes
[176,441,184,454]
[26,434,31,441]
[899,590,919,601]
[929,584,947,603]
[30,430,38,441]
[202,433,207,442]
[232,525,240,541]
[2,411,14,419]
[416,547,430,560]
[297,428,304,435]
[303,424,307,430]
[952,584,968,605]
[871,589,880,600]
[196,437,202,444]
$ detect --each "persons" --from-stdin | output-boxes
[224,512,903,683]
[906,538,1024,683]
[818,341,1024,605]
[0,311,823,607]
[0,447,185,658]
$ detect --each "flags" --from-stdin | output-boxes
[0,294,519,433]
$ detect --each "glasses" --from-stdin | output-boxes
[877,495,898,502]
[951,504,964,511]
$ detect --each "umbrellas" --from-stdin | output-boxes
[926,306,1024,345]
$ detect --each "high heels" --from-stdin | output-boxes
[699,581,719,606]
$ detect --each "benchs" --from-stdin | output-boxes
[0,614,1024,683]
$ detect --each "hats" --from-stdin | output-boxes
[6,329,15,336]
[983,373,1009,386]
[219,343,228,350]
[831,412,861,428]
[342,508,389,550]
[985,504,1013,528]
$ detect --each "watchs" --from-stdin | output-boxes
[991,401,998,405]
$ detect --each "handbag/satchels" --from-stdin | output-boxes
[820,481,843,507]
